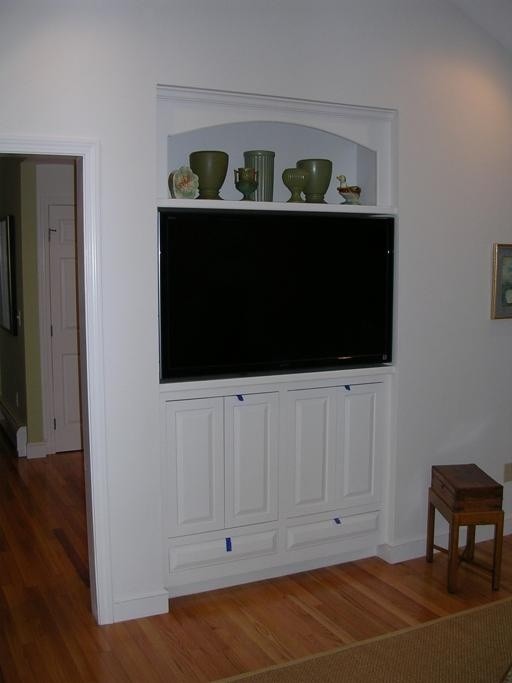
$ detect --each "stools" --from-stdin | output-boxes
[427,488,504,592]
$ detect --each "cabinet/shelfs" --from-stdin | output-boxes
[160,364,395,598]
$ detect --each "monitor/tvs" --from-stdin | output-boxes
[156,206,396,384]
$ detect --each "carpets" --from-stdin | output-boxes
[211,597,512,683]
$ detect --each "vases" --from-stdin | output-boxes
[190,151,332,204]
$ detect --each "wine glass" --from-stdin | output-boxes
[189,151,229,200]
[282,168,309,203]
[296,158,332,203]
[233,165,260,201]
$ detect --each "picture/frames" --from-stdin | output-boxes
[491,243,512,319]
[0,215,17,336]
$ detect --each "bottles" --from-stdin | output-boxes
[243,150,275,202]
